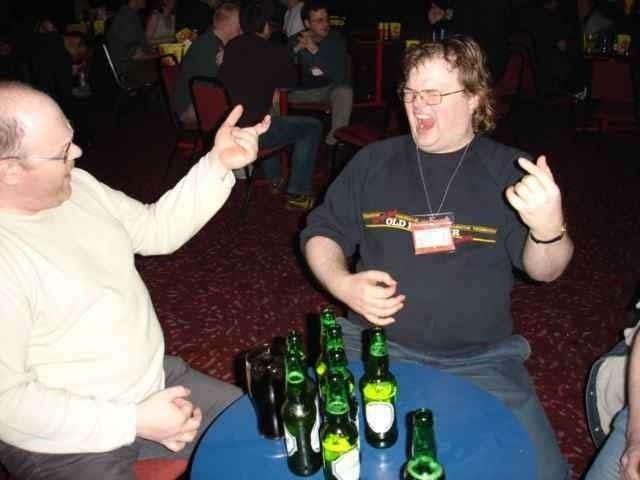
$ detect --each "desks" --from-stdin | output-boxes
[191,359,538,479]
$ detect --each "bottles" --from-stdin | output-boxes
[599,31,609,56]
[401,406,447,480]
[280,306,397,480]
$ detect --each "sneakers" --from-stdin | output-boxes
[272,176,317,210]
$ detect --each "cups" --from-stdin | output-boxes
[618,34,630,50]
[243,341,285,442]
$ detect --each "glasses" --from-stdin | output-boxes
[1,142,70,165]
[397,86,465,106]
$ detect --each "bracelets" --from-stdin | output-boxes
[529,222,568,246]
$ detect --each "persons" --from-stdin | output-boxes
[1,1,639,213]
[299,31,576,480]
[0,81,271,480]
[581,325,640,480]
